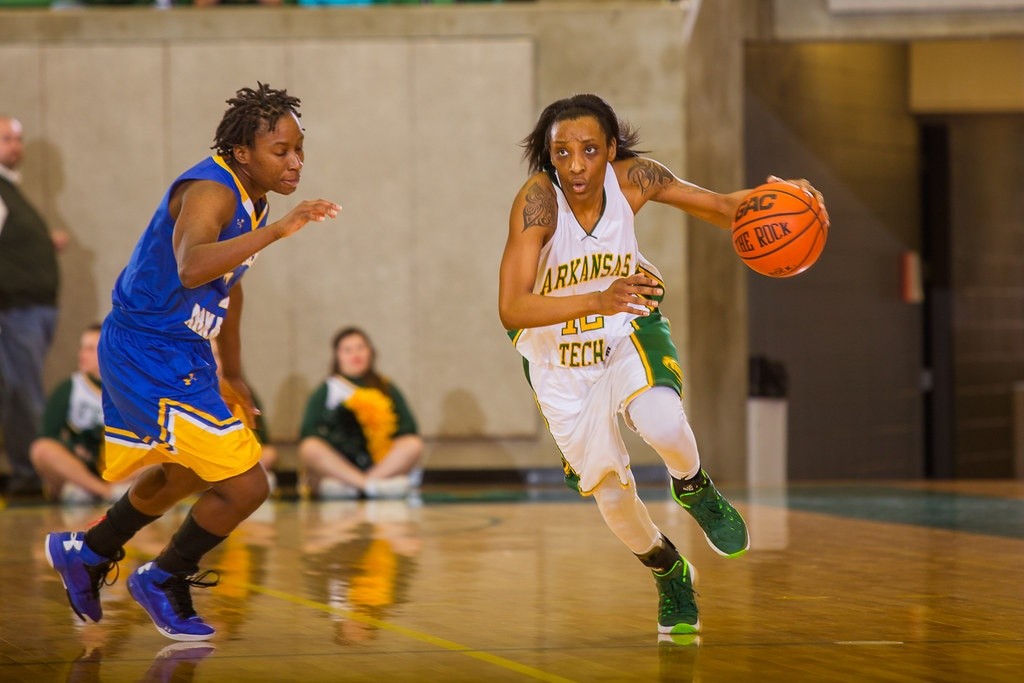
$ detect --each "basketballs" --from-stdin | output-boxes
[729,181,828,278]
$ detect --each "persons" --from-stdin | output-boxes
[44,79,342,641]
[296,327,424,501]
[162,323,280,503]
[497,93,830,634]
[0,113,69,505]
[56,501,425,652]
[28,322,139,508]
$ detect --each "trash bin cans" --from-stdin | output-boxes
[745,360,788,491]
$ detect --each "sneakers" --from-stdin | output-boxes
[657,634,702,683]
[651,554,700,633]
[126,559,220,642]
[671,469,751,558]
[143,641,216,683]
[45,532,125,624]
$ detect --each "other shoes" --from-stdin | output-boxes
[317,477,358,498]
[59,482,93,508]
[371,475,411,498]
[110,482,135,502]
